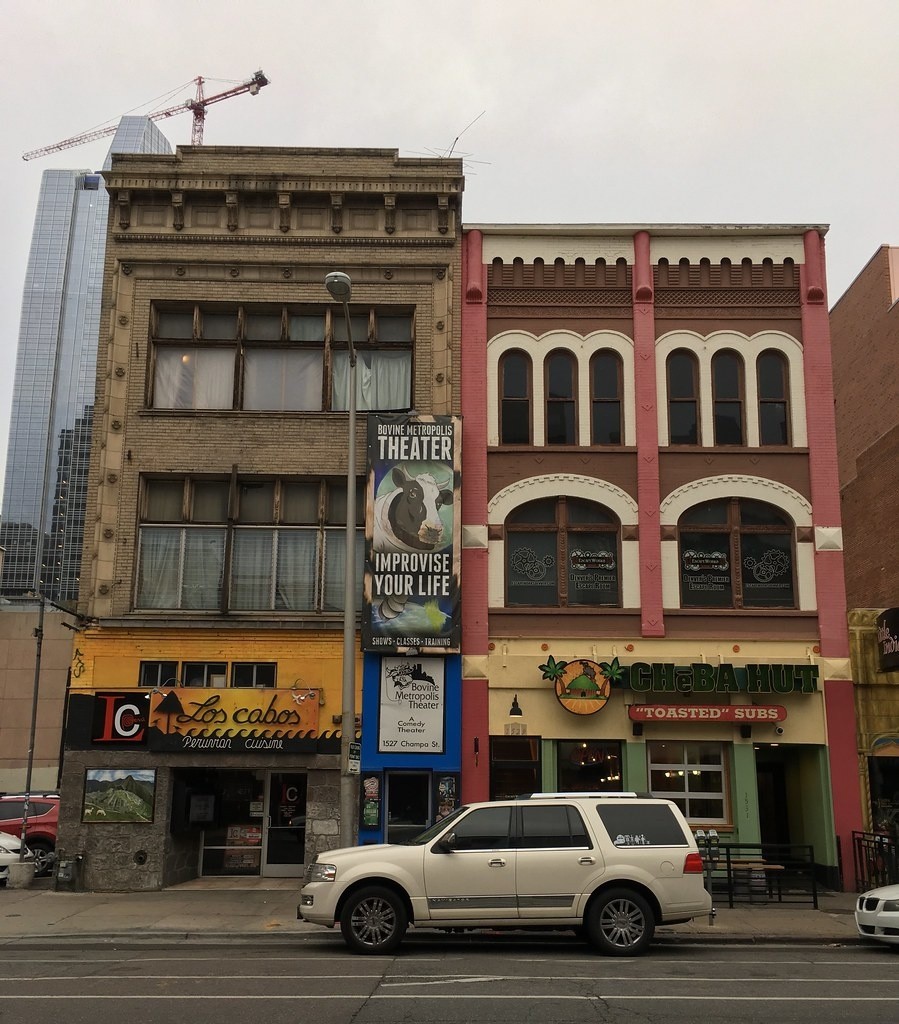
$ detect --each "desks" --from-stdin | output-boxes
[704,862,785,900]
[701,857,772,897]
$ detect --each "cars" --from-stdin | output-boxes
[0,829,36,887]
[855,880,899,944]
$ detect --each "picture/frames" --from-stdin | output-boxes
[81,766,157,825]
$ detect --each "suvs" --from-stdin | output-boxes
[0,790,59,878]
[295,789,714,959]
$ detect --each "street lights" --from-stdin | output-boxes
[321,267,363,855]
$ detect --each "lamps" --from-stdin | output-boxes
[509,693,522,717]
[145,687,167,699]
[291,676,316,705]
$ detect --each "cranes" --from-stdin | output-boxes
[23,65,270,168]
